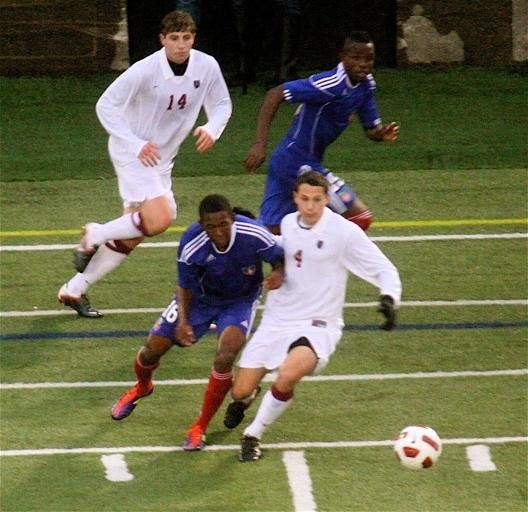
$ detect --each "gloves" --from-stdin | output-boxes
[376,295,396,331]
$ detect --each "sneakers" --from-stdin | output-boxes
[183,428,207,452]
[223,384,261,429]
[72,222,99,273]
[239,434,263,462]
[110,382,154,420]
[57,283,104,319]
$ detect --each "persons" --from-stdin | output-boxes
[224,169,402,462]
[243,31,400,234]
[111,193,284,451]
[57,10,233,318]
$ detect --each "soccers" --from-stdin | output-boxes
[395,425,441,471]
